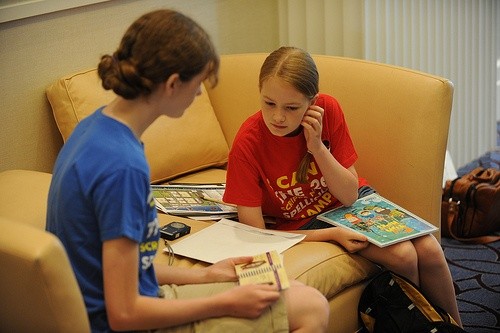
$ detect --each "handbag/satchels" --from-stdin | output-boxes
[441,165,500,243]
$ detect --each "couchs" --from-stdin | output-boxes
[0,52,454,333]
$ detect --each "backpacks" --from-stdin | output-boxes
[358,271,467,333]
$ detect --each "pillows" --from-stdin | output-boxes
[46,66,231,185]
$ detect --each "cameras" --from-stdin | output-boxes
[159,221,191,241]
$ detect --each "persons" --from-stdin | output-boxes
[44,10,329,333]
[222,47,464,330]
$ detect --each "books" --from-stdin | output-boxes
[316,194,439,249]
